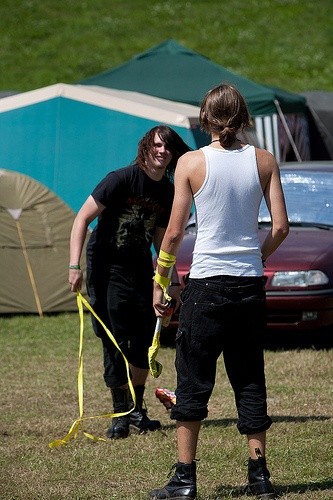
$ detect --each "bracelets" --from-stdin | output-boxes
[68,265,80,269]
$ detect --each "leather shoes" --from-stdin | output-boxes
[150,462,197,500]
[244,458,274,497]
[130,409,161,434]
[107,410,129,439]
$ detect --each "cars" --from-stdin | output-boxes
[153,159,332,347]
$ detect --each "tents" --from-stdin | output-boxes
[0,83,211,256]
[0,169,92,314]
[72,38,333,162]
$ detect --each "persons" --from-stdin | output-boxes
[149,81,288,500]
[68,125,193,438]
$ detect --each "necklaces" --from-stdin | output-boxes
[206,140,220,145]
